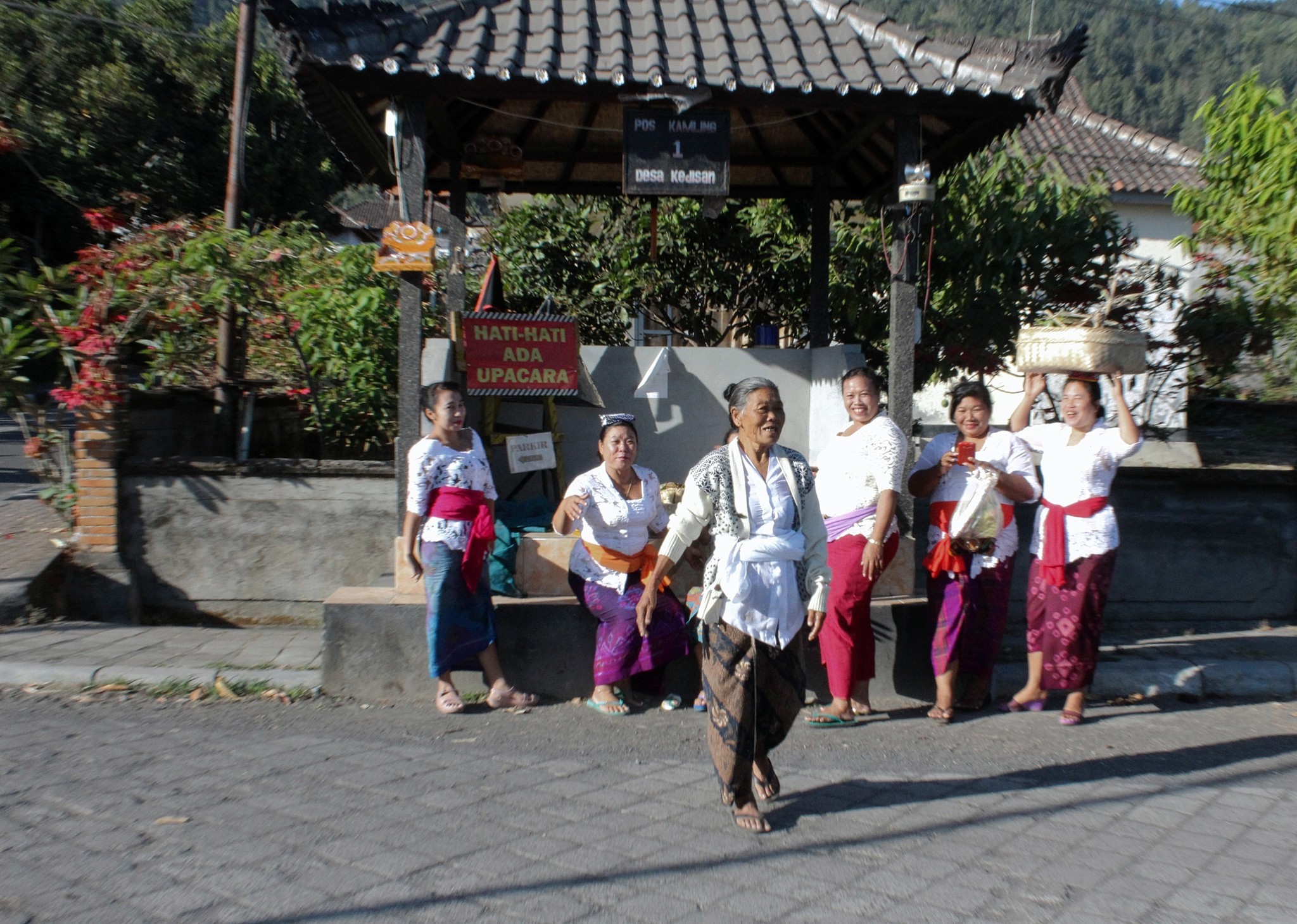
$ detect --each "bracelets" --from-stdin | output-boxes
[868,538,885,547]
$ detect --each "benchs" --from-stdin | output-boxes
[321,579,930,697]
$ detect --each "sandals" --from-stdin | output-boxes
[434,690,462,713]
[487,681,540,708]
[693,691,708,713]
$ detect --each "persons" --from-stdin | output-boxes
[638,376,832,832]
[907,381,1042,721]
[693,426,738,711]
[997,369,1143,725]
[802,363,907,725]
[550,421,690,716]
[403,382,541,713]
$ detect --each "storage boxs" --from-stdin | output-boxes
[514,532,580,597]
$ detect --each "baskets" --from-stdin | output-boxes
[1017,323,1147,374]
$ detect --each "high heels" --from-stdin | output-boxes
[1060,707,1083,728]
[999,694,1046,715]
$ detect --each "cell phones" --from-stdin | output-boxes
[957,442,975,466]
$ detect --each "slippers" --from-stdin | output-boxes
[724,791,773,835]
[953,692,992,714]
[927,702,951,724]
[614,688,643,708]
[751,757,780,801]
[588,690,641,718]
[853,704,874,717]
[806,712,858,728]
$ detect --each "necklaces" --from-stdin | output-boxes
[625,472,634,500]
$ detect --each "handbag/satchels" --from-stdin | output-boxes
[949,463,1005,538]
